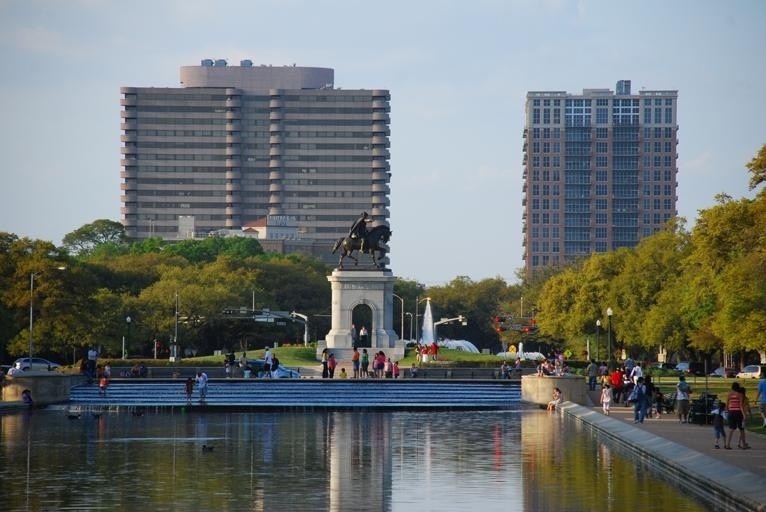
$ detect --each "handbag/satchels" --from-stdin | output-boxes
[627,392,637,403]
[678,382,689,392]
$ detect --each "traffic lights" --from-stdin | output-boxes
[529,319,537,324]
[524,326,537,332]
[496,327,506,331]
[496,316,505,322]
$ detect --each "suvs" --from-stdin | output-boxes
[230,360,301,378]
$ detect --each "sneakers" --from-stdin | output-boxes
[633,415,687,424]
[714,444,752,449]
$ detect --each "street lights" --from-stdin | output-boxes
[417,297,431,343]
[126,315,131,359]
[406,311,413,343]
[607,307,613,361]
[29,264,66,369]
[597,319,600,361]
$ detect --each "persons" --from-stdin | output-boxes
[271,353,278,378]
[79,336,172,378]
[372,341,439,380]
[263,346,271,378]
[361,349,370,378]
[351,324,356,347]
[339,368,347,378]
[328,353,337,378]
[349,211,369,254]
[224,348,237,379]
[351,347,359,379]
[194,372,208,403]
[359,326,368,347]
[321,348,329,379]
[98,376,109,396]
[500,353,766,450]
[184,377,195,405]
[239,352,254,378]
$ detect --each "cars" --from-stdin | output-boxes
[13,357,62,370]
[654,361,766,379]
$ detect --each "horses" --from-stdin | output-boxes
[331,225,391,269]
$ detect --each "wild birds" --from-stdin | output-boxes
[203,445,214,452]
[68,414,81,420]
[92,412,104,419]
